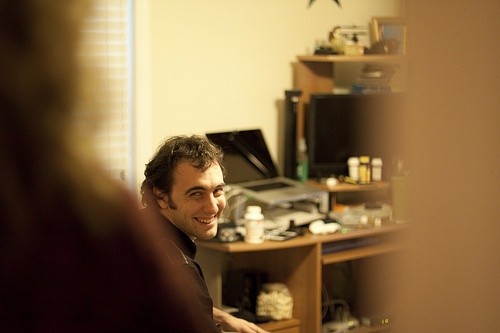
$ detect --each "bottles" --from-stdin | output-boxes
[347,157,359,179]
[359,156,370,184]
[244,206,265,244]
[371,157,382,182]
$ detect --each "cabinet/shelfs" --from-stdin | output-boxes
[291,53,409,212]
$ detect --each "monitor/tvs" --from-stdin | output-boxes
[307,94,400,178]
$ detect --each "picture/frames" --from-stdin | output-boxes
[370,16,406,56]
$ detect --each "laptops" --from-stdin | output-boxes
[205,129,326,206]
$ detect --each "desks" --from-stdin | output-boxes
[191,193,412,333]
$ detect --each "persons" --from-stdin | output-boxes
[0,0,214,333]
[139,134,268,333]
